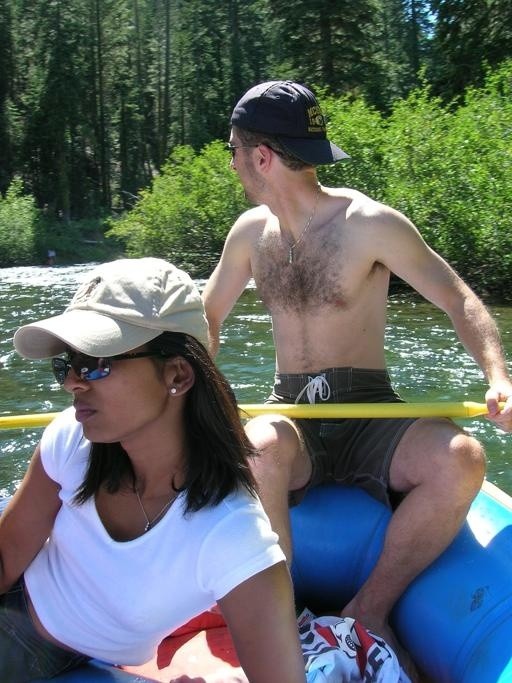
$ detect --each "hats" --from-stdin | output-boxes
[13,256,210,361]
[230,79,353,166]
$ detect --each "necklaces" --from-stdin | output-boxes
[277,182,322,263]
[134,485,177,531]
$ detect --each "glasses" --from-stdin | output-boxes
[227,141,284,159]
[51,351,160,385]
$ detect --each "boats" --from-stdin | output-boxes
[49,431,512,682]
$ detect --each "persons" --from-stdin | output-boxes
[195,80,512,682]
[0,256,310,682]
